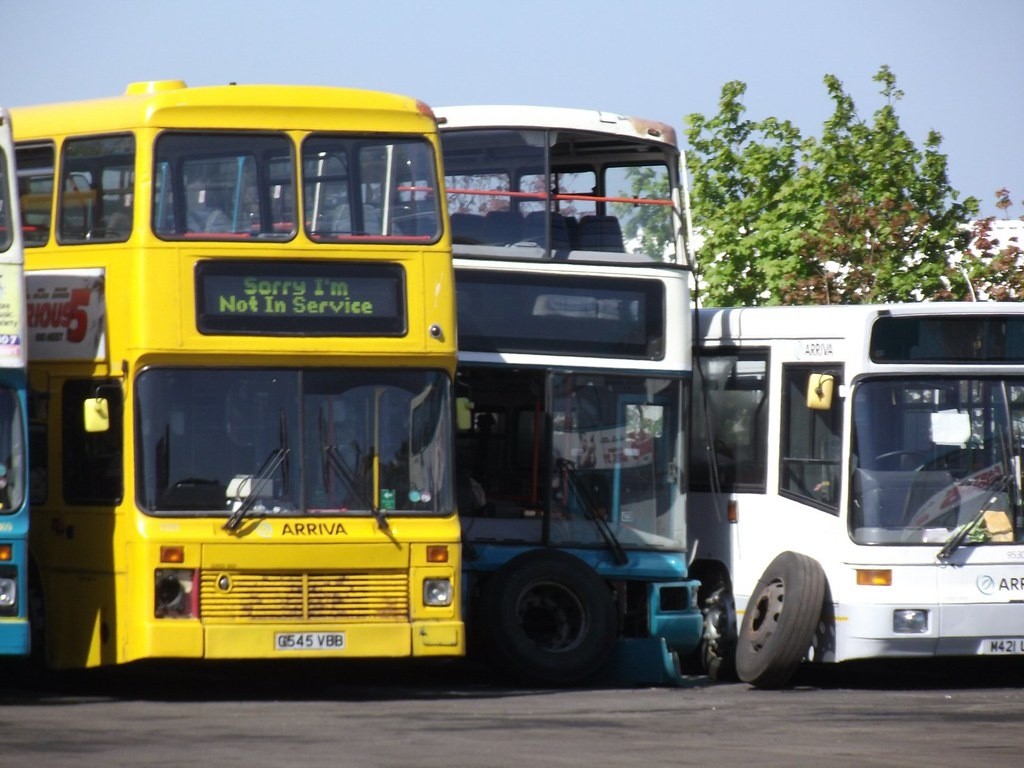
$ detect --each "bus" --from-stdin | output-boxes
[0,77,482,687]
[328,102,705,685]
[601,297,1023,692]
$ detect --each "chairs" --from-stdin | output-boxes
[450,209,625,248]
[83,194,387,240]
